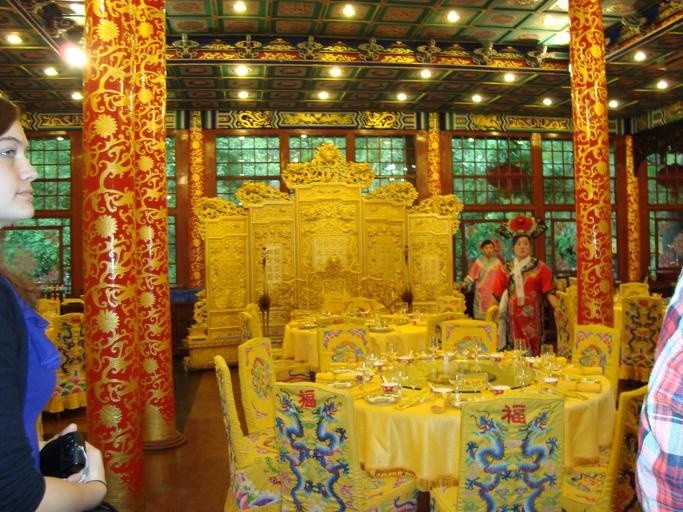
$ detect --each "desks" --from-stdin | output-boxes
[316,352,613,512]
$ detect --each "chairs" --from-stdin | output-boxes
[429,395,566,511]
[570,322,621,396]
[213,354,282,512]
[236,279,666,385]
[237,337,279,434]
[271,380,417,512]
[33,298,87,414]
[562,384,649,511]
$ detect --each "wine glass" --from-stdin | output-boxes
[305,301,421,329]
[328,336,556,409]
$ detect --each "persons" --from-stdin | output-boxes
[0,96,107,512]
[452,240,504,351]
[488,234,566,358]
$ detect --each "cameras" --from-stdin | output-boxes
[40,431,85,478]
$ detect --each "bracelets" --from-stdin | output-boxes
[86,479,108,489]
